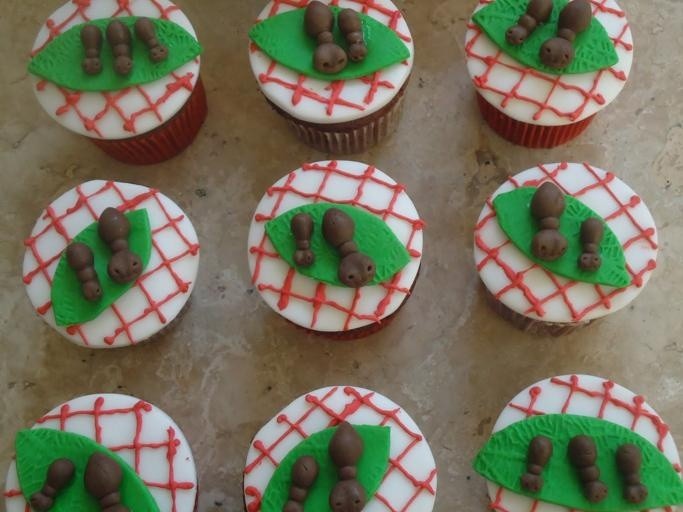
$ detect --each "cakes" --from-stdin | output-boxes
[21,178,200,350]
[3,392,199,512]
[247,160,429,341]
[242,385,439,511]
[464,1,635,149]
[25,1,209,165]
[471,374,682,511]
[474,162,659,338]
[246,0,415,154]
